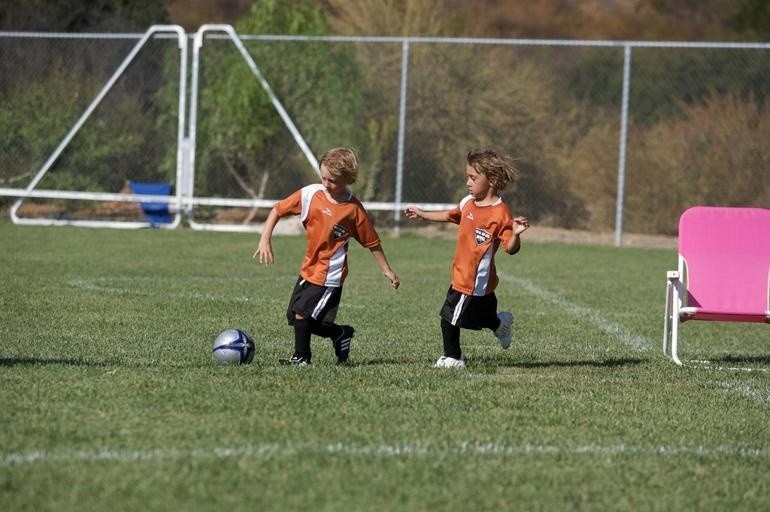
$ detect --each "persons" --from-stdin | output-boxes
[251,145,401,366]
[404,148,530,368]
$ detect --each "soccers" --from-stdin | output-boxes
[212,327,255,365]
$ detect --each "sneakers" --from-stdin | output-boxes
[431,355,467,369]
[278,355,312,366]
[334,325,354,365]
[494,312,513,349]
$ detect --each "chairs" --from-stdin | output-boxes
[663,206,769,367]
